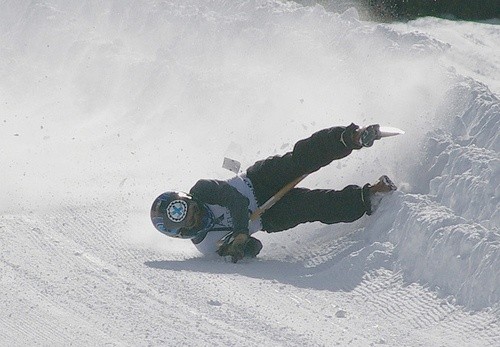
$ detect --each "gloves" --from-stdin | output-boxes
[216,236,262,264]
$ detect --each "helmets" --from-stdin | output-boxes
[151,191,208,240]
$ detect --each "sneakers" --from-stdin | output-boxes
[363,175,397,212]
[340,123,381,152]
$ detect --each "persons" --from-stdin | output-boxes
[150,122,397,264]
